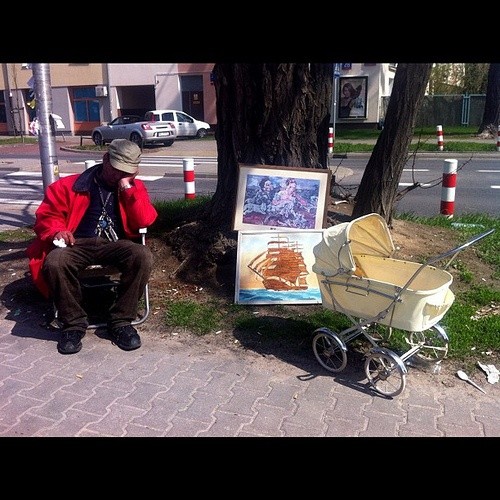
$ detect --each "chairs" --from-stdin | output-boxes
[53,159,149,329]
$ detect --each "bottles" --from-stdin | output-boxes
[395,351,442,374]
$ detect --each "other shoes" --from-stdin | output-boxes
[58,329,86,353]
[108,324,142,349]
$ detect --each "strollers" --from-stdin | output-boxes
[310,212,496,397]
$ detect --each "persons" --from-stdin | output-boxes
[34,140,159,354]
[339,83,361,117]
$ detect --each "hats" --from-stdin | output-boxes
[109,139,141,173]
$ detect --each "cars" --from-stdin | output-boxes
[92,115,176,149]
[145,110,211,138]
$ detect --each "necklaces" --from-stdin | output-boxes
[95,178,119,242]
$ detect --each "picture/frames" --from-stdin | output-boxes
[234,228,327,305]
[338,75,368,121]
[231,162,332,233]
